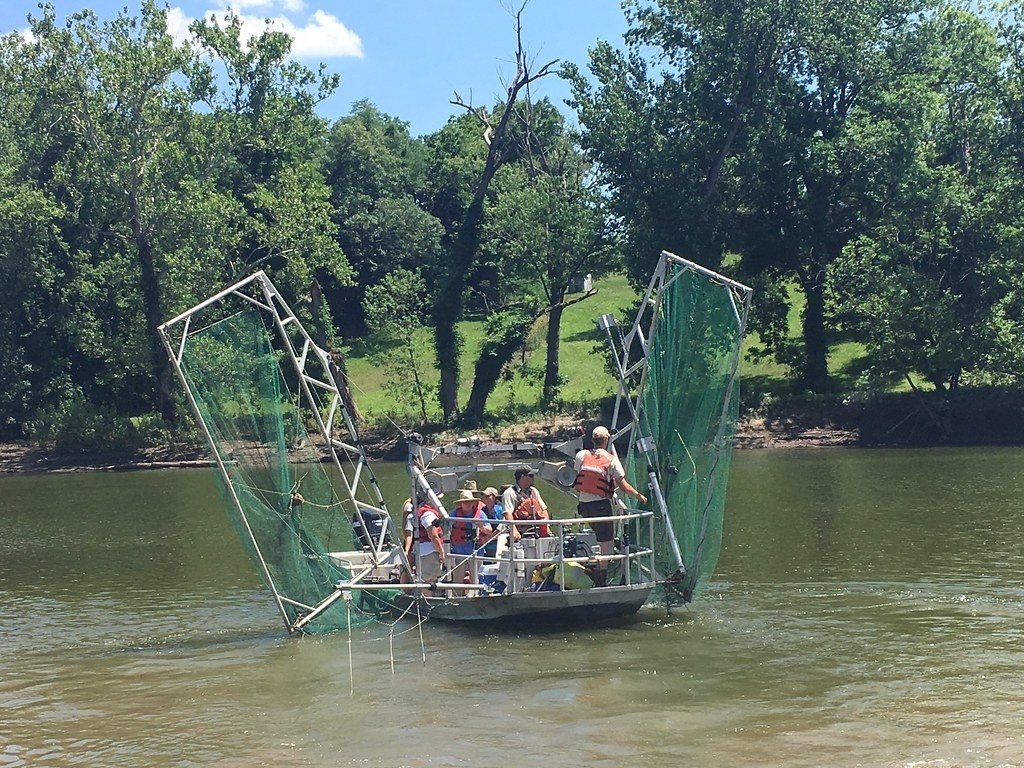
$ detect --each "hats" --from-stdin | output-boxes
[515,465,540,475]
[454,491,481,502]
[482,487,501,497]
[593,426,611,438]
[457,480,480,492]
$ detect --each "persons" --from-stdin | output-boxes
[501,465,552,542]
[415,491,445,597]
[401,480,514,597]
[573,425,648,587]
[447,490,492,596]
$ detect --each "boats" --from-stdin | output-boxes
[303,432,659,622]
